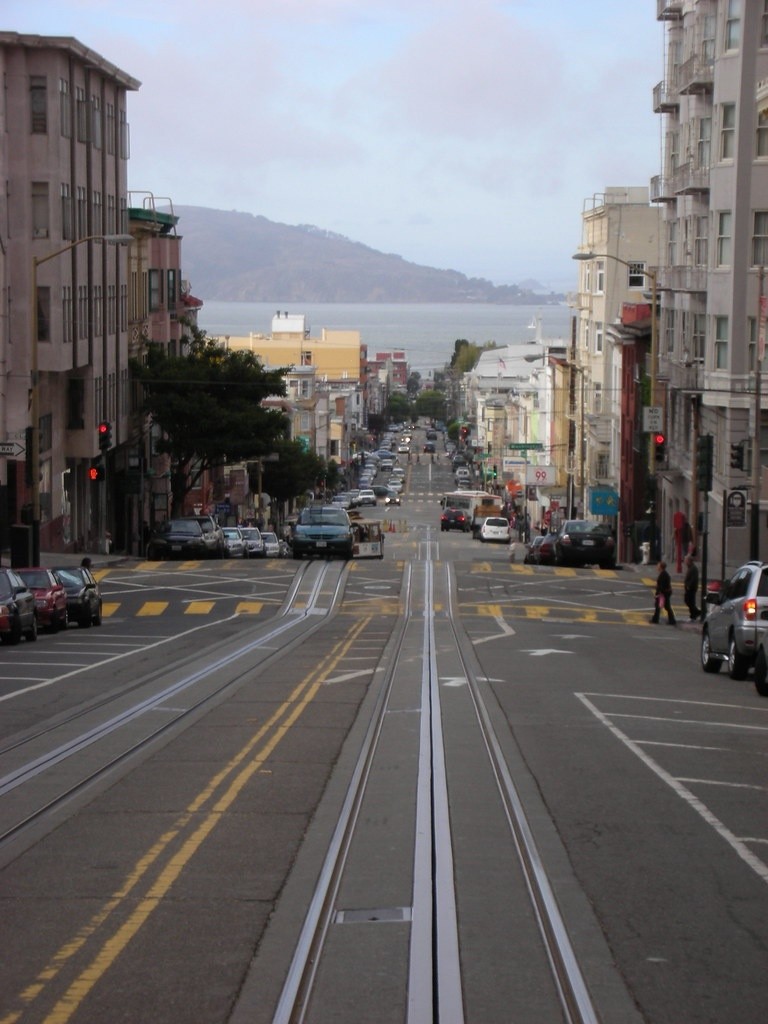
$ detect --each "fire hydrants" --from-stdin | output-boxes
[640,540,649,566]
[104,533,112,553]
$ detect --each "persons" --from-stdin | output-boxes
[142,521,168,559]
[643,519,660,564]
[684,555,701,623]
[81,558,92,569]
[506,508,548,537]
[237,513,275,532]
[648,561,676,625]
[395,453,440,464]
[321,488,337,505]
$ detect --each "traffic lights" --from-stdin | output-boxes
[100,422,111,451]
[729,444,744,469]
[463,428,468,436]
[90,466,102,482]
[654,433,664,462]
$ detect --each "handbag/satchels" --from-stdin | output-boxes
[659,594,664,608]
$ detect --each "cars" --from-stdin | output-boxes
[525,520,616,568]
[146,506,353,561]
[328,418,412,509]
[0,565,103,644]
[423,419,472,492]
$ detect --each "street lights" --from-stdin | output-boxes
[573,251,658,565]
[33,233,136,568]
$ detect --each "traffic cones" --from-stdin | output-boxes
[389,522,396,533]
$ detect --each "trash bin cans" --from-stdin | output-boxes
[11,525,34,567]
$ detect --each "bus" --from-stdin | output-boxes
[440,491,504,528]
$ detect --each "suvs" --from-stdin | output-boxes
[702,559,768,679]
[478,517,511,543]
[442,508,470,533]
